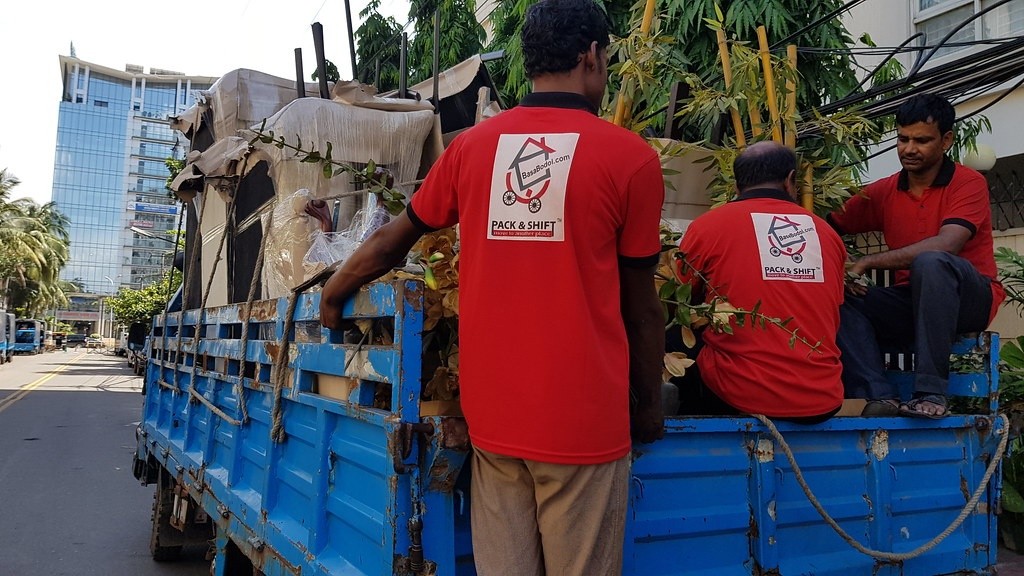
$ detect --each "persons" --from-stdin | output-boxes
[671,140,848,426]
[825,93,1008,420]
[318,0,666,576]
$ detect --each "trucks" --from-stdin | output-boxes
[0,310,17,364]
[128,250,1011,576]
[12,317,47,355]
[114,325,149,376]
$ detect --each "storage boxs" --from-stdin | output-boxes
[646,138,719,220]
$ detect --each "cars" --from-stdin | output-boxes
[67,334,87,348]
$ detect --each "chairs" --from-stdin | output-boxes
[295,0,507,193]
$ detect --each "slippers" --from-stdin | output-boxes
[896,394,948,417]
[859,400,900,418]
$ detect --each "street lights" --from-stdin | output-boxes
[104,274,122,338]
